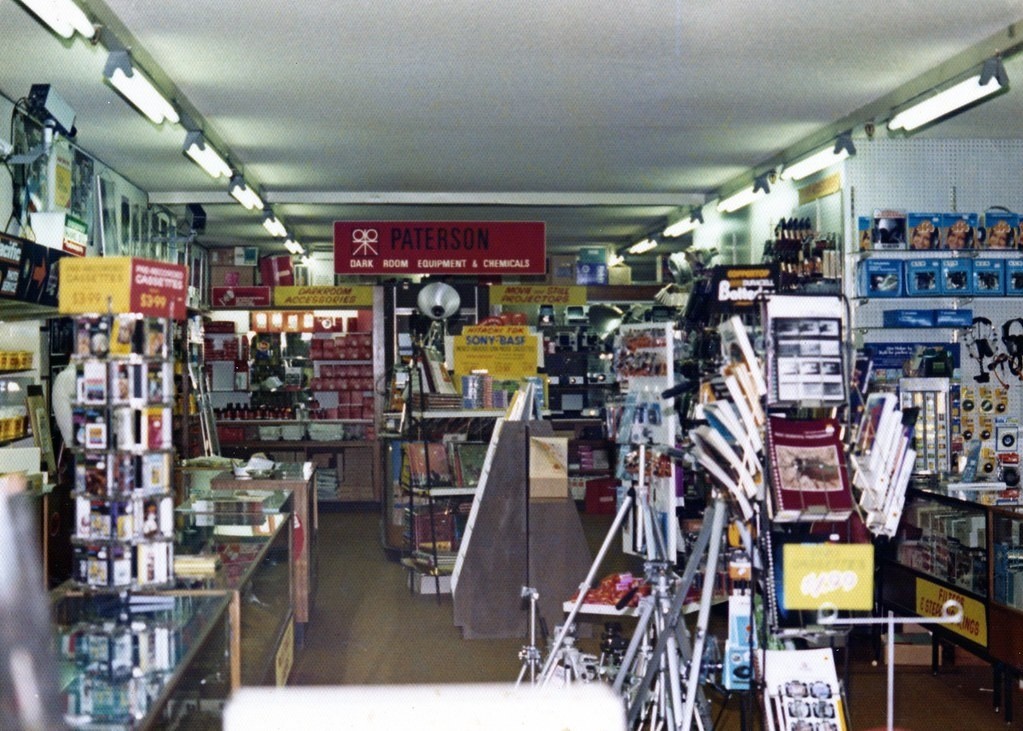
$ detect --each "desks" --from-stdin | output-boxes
[210,461,321,636]
[45,487,297,731]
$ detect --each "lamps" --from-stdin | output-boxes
[778,134,857,182]
[12,0,101,48]
[228,176,266,213]
[181,131,234,181]
[98,51,183,129]
[260,211,287,240]
[662,209,704,239]
[280,232,305,256]
[607,253,626,268]
[629,232,659,256]
[886,58,1011,141]
[716,178,771,213]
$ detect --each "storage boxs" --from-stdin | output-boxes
[311,378,321,391]
[310,337,324,350]
[347,316,358,332]
[300,312,317,331]
[334,336,345,346]
[321,364,373,420]
[324,350,336,360]
[851,206,1023,298]
[335,347,345,360]
[346,347,358,360]
[346,334,360,346]
[310,349,322,361]
[314,317,334,331]
[251,311,267,331]
[324,338,334,349]
[360,334,371,345]
[359,347,371,361]
[358,309,373,332]
[268,312,283,332]
[283,312,301,332]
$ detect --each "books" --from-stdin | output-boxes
[170,543,219,577]
[400,505,470,575]
[686,312,921,731]
[401,440,491,488]
[411,374,508,413]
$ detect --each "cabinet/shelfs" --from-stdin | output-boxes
[400,362,552,595]
[208,314,385,508]
[537,323,620,510]
